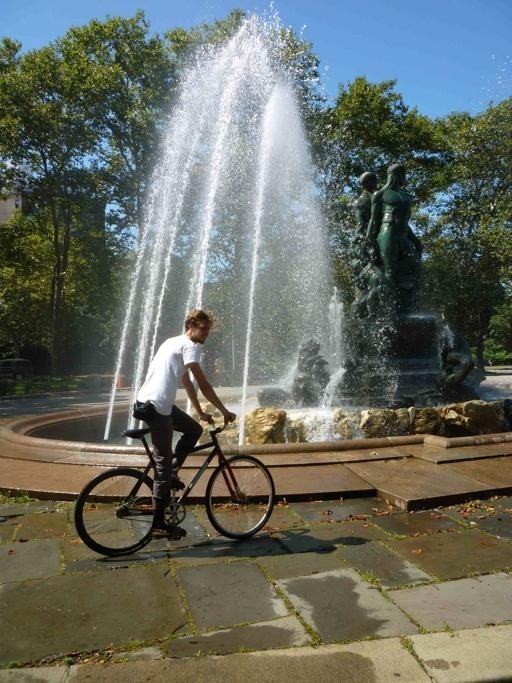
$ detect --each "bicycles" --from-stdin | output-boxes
[74,417,276,557]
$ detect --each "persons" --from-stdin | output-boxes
[346,162,426,320]
[131,308,236,540]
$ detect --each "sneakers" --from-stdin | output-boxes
[170,478,185,490]
[152,527,187,540]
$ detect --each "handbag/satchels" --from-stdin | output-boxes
[133,400,154,422]
